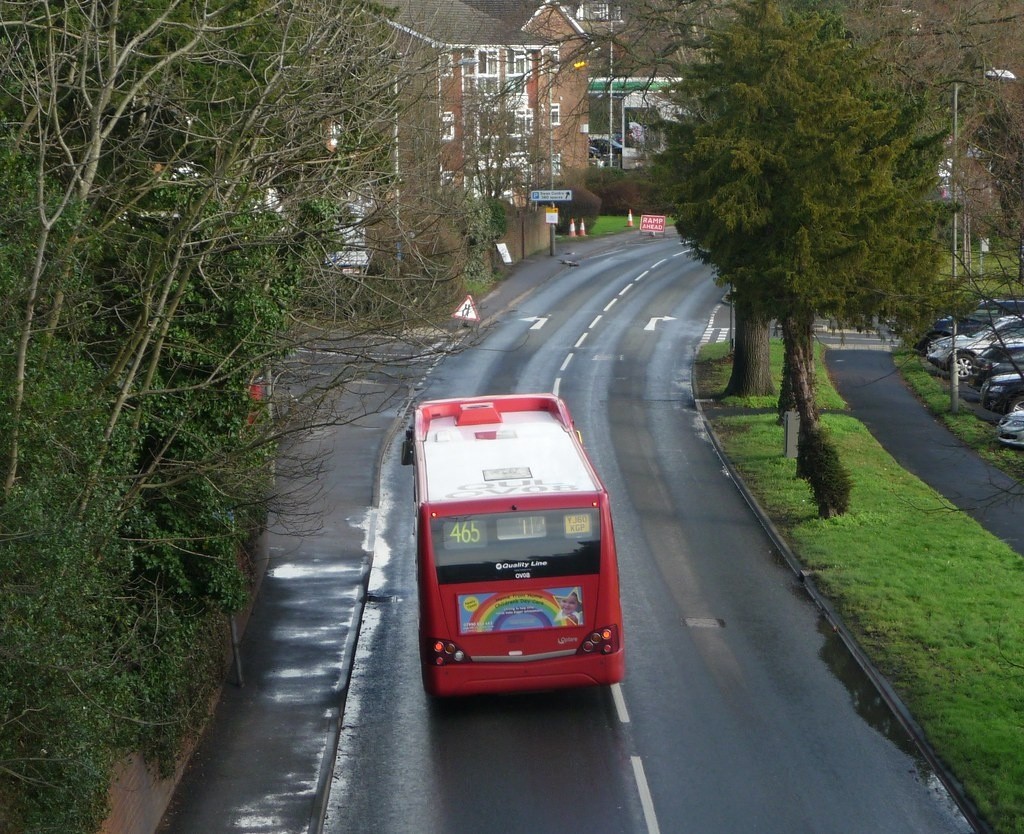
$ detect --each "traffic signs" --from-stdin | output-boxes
[530,189,572,201]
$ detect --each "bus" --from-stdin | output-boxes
[399,392,627,700]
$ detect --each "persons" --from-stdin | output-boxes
[557,592,580,624]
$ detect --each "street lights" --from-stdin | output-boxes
[577,217,587,236]
[951,69,1016,416]
[624,209,633,227]
[567,218,577,238]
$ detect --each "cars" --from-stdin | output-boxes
[913,295,1024,414]
[997,410,1024,448]
[588,131,635,171]
